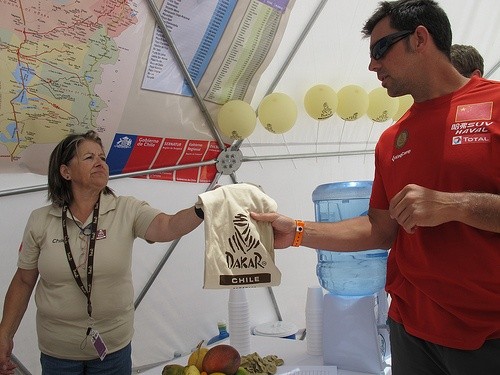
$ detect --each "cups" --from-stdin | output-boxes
[305,288,324,359]
[227,288,251,355]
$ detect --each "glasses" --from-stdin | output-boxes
[370,29,415,61]
[75,235,88,272]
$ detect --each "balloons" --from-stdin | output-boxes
[305,85,338,120]
[366,87,398,121]
[258,93,298,134]
[335,85,369,121]
[218,100,256,139]
[393,94,414,122]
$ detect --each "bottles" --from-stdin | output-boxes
[311,180,390,295]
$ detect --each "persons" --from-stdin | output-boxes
[248,1,500,375]
[1,130,206,375]
[449,44,484,79]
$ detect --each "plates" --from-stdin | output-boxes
[253,319,299,338]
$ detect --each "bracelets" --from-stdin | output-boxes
[193,205,205,221]
[292,220,304,247]
